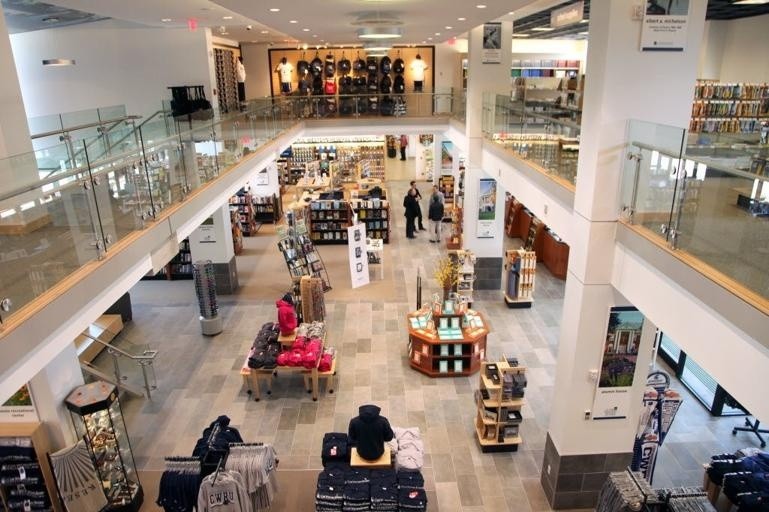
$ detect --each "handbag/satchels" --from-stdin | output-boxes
[297,51,406,119]
[171,86,214,121]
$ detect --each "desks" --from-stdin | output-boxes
[365,237,386,286]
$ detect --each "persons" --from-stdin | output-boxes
[275,57,293,93]
[429,185,445,233]
[403,189,419,239]
[409,181,426,233]
[609,359,618,383]
[276,293,298,336]
[398,135,408,160]
[428,195,444,243]
[236,56,246,101]
[348,405,394,460]
[630,341,636,353]
[410,53,428,93]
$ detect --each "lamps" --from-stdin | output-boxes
[39,17,76,68]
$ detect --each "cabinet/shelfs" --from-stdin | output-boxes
[277,230,336,297]
[438,167,568,310]
[513,61,580,82]
[65,378,143,509]
[241,273,337,403]
[404,302,493,379]
[474,355,527,456]
[692,82,767,136]
[141,184,289,282]
[277,143,393,246]
[0,420,60,511]
